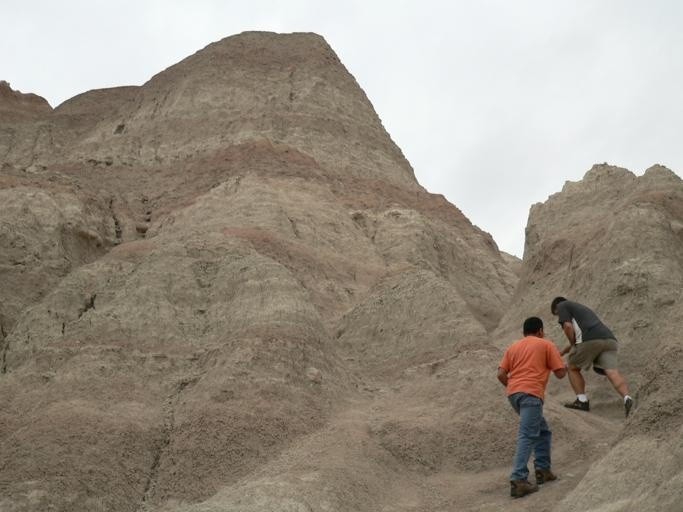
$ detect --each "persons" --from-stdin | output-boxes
[495,316,567,497]
[549,296,632,415]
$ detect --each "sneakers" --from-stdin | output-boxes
[509,478,539,497]
[624,398,633,417]
[534,469,557,484]
[564,397,589,411]
